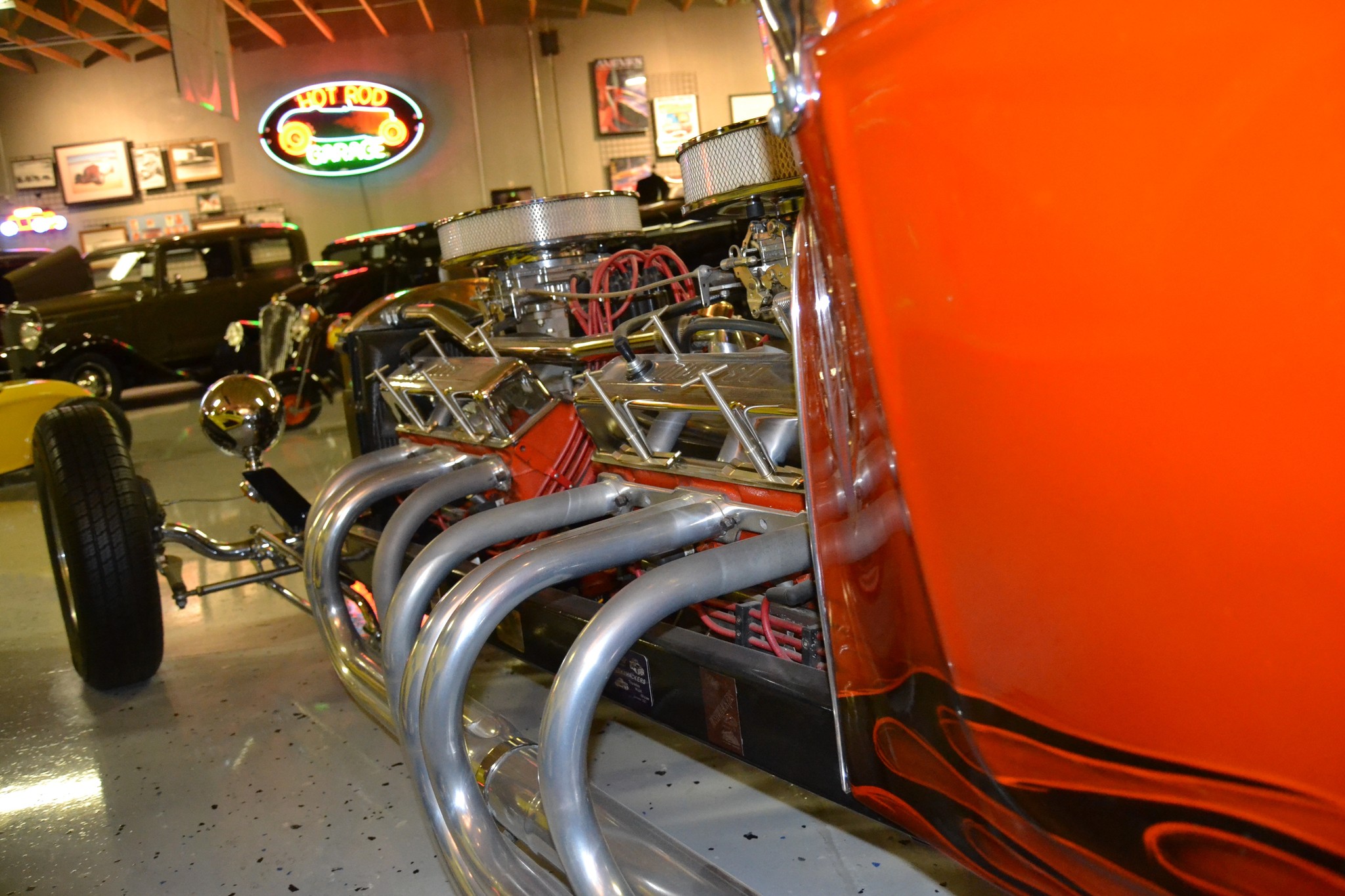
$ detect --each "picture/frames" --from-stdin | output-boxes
[52,137,138,207]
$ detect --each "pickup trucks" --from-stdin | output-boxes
[0,219,444,479]
